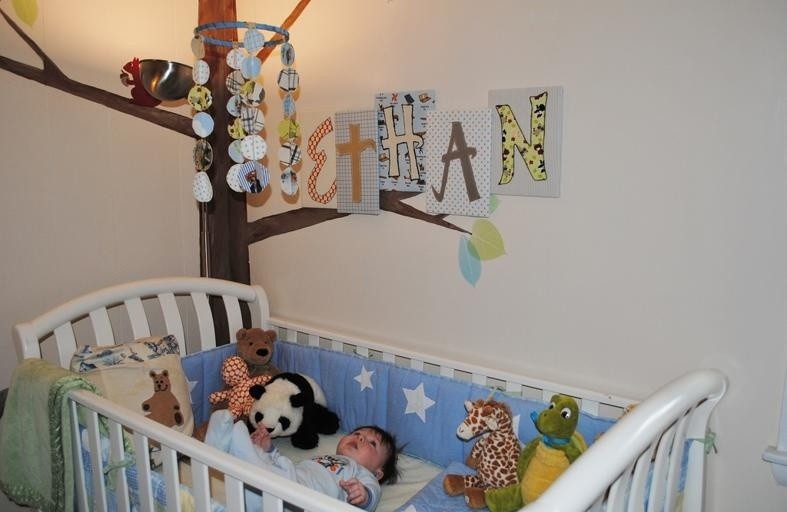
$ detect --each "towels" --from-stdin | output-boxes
[0,359,131,512]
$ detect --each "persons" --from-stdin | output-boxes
[203,409,409,512]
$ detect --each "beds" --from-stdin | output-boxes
[12,277,731,512]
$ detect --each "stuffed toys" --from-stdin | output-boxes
[246,371,339,451]
[444,394,522,509]
[208,326,282,423]
[208,353,273,422]
[482,392,588,512]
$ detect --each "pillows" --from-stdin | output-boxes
[60,327,198,477]
